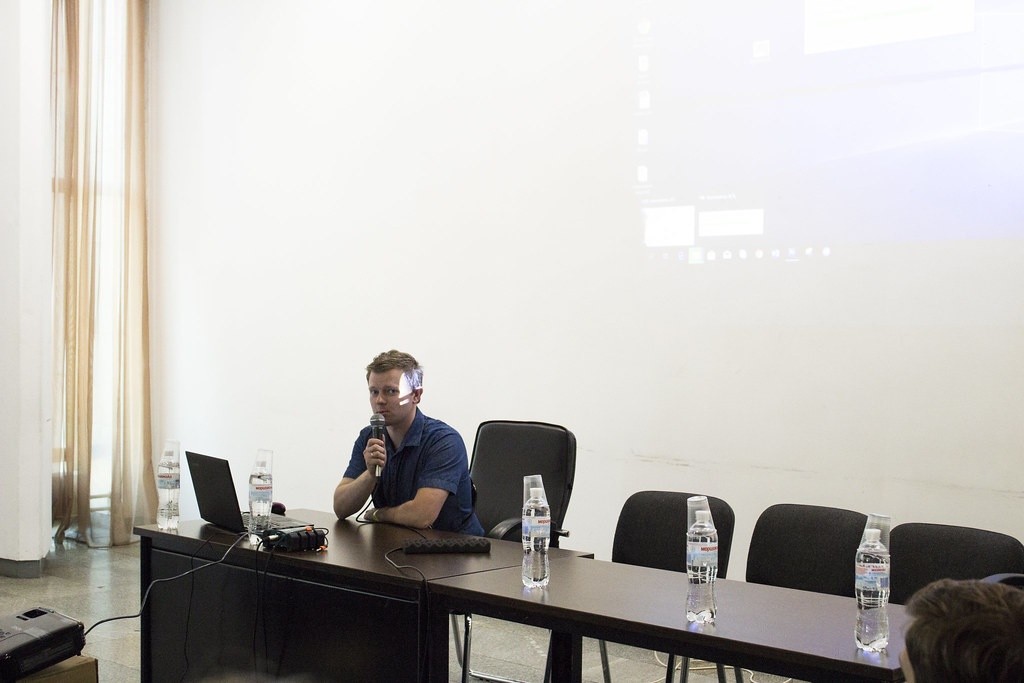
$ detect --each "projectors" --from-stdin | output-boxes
[0,607,86,683]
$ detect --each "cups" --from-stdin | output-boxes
[252,449,278,475]
[161,438,181,469]
[686,496,717,537]
[522,475,550,509]
[857,512,892,558]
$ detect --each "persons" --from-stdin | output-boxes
[332,350,485,537]
[898,580,1024,683]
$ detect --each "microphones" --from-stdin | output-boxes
[370,413,386,485]
[264,526,317,544]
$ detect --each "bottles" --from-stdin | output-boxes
[522,488,550,588]
[686,510,719,623]
[156,450,180,530]
[854,529,889,653]
[248,461,273,547]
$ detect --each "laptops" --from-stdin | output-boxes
[185,451,314,533]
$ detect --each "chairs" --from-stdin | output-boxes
[598,490,735,683]
[889,523,1024,605]
[450,420,577,683]
[681,503,868,683]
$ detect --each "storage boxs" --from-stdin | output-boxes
[16,654,98,683]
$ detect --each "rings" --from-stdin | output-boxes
[370,453,375,458]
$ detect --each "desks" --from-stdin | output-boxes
[132,507,594,683]
[425,556,909,683]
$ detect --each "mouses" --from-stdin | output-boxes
[271,502,286,513]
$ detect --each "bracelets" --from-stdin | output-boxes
[373,508,380,522]
